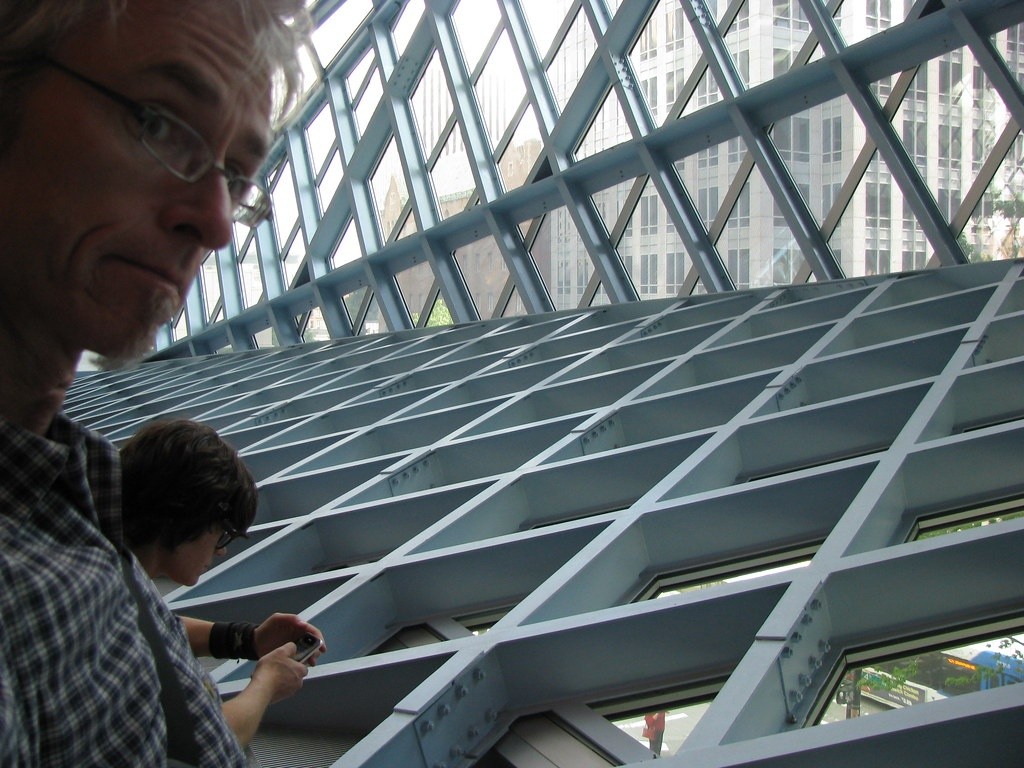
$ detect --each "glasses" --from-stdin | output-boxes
[33,51,274,222]
[213,521,240,548]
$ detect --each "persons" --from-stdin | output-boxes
[644,712,664,756]
[121,419,327,768]
[0,0,307,768]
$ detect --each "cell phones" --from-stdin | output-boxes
[290,631,323,663]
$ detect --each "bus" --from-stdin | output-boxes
[838,646,1024,710]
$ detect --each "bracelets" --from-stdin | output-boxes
[209,622,257,659]
